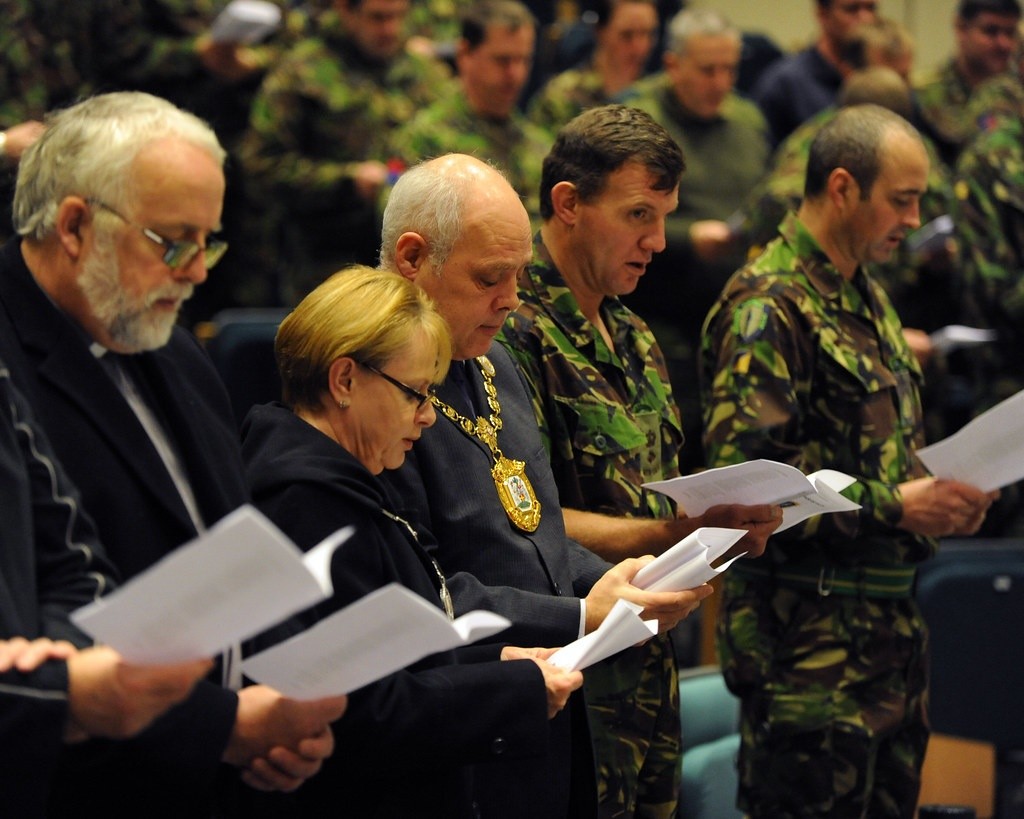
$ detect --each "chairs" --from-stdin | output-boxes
[675,665,751,819]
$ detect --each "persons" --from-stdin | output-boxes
[700,104,999,819]
[0,0,1024,358]
[0,92,783,819]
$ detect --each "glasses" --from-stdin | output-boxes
[85,198,228,268]
[357,359,437,411]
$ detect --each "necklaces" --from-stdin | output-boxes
[382,507,455,621]
[430,354,542,532]
